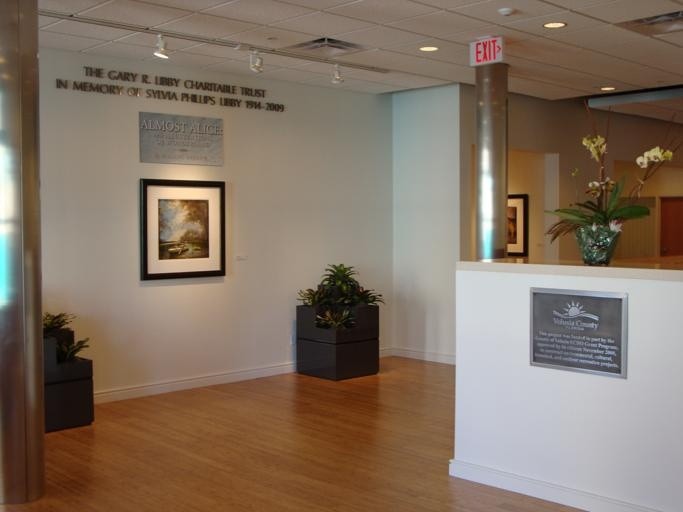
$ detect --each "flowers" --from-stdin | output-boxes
[545,99,683,243]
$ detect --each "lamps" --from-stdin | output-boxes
[38,9,393,84]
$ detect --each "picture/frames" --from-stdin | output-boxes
[140,177,227,279]
[506,193,529,258]
[530,288,631,380]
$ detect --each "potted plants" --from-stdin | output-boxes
[42,310,95,434]
[296,263,385,380]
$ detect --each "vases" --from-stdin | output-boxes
[574,227,621,267]
